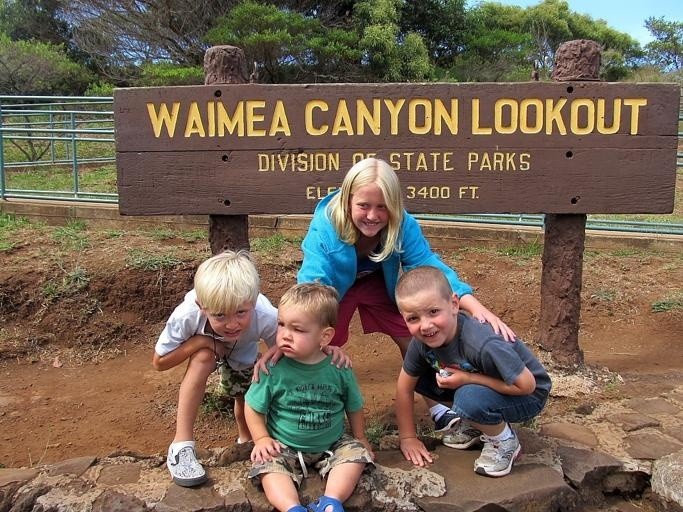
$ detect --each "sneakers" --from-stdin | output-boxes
[441,420,483,449]
[433,408,461,432]
[473,426,524,477]
[166,441,208,489]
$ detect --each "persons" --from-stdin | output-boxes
[394,266,552,478]
[243,283,373,511]
[153,249,352,488]
[249,157,517,385]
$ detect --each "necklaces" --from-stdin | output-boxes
[210,330,237,374]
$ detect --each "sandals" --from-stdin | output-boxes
[286,494,345,512]
